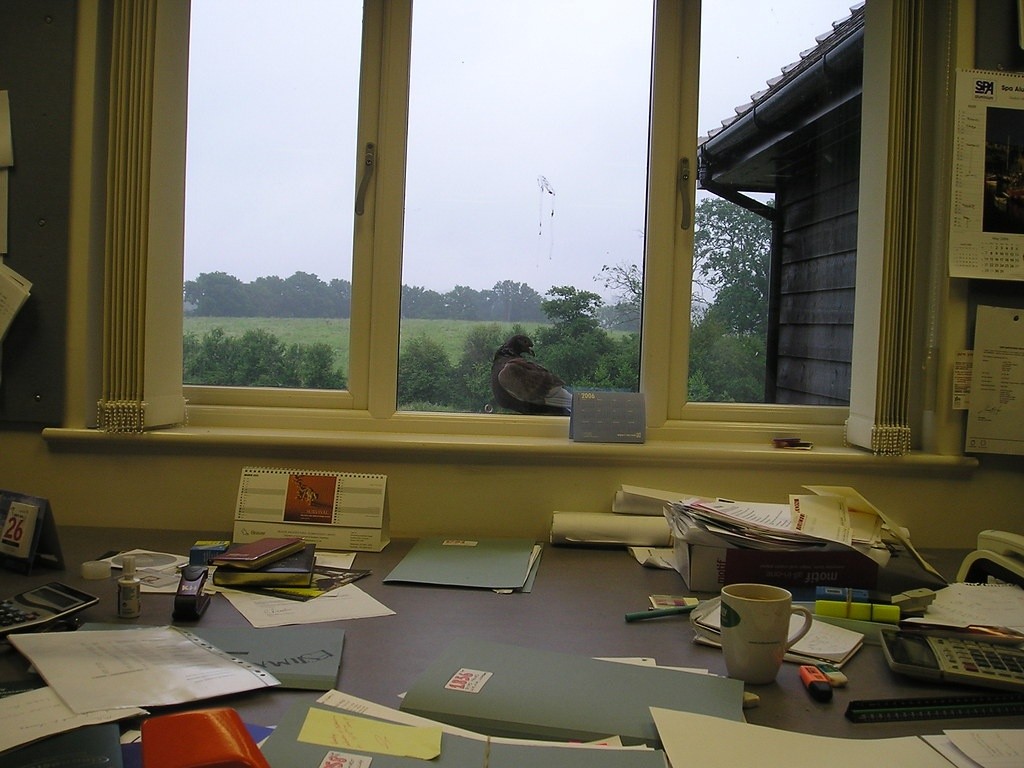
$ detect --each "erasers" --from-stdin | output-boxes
[817,663,848,686]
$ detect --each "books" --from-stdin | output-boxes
[0,621,1024,767]
[207,538,317,589]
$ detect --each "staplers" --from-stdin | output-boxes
[172,565,211,620]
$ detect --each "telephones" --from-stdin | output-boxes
[955,530,1024,582]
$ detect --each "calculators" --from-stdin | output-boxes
[0,581,100,634]
[879,627,1024,692]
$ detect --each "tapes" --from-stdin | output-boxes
[82,560,111,579]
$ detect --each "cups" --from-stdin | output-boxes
[721,584,813,685]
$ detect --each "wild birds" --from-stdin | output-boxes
[491,335,573,416]
